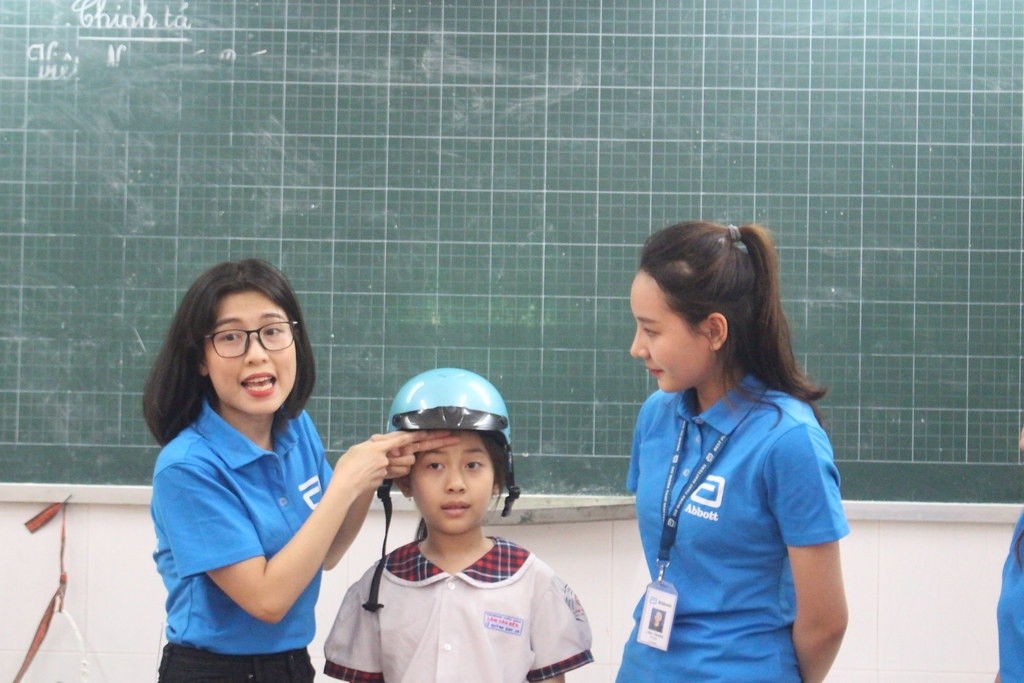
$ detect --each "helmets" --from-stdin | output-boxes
[386,368,513,475]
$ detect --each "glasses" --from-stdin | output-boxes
[204,321,299,358]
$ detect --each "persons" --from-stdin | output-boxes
[142,259,460,683]
[615,221,849,683]
[323,368,595,683]
[993,430,1024,683]
[649,610,664,633]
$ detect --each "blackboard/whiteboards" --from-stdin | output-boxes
[0,0,1024,510]
